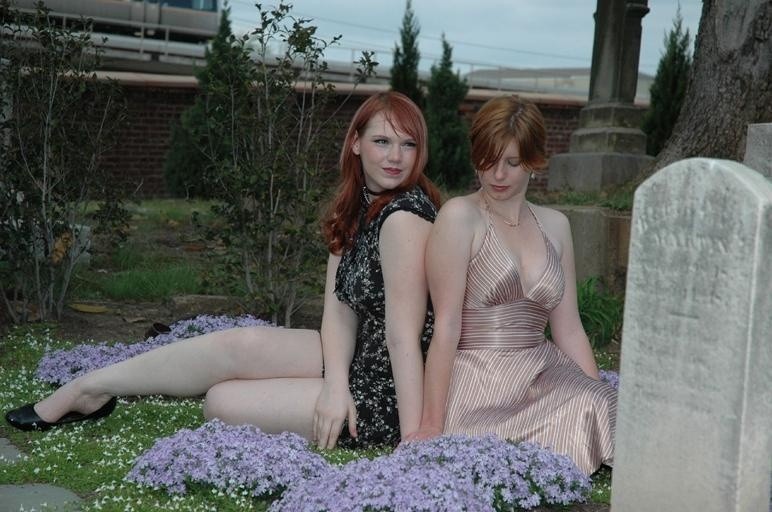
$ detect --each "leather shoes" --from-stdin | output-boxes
[5,397,116,430]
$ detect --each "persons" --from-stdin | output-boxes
[5,92,442,449]
[410,94,620,476]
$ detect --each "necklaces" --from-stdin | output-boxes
[479,190,523,228]
[362,185,382,197]
